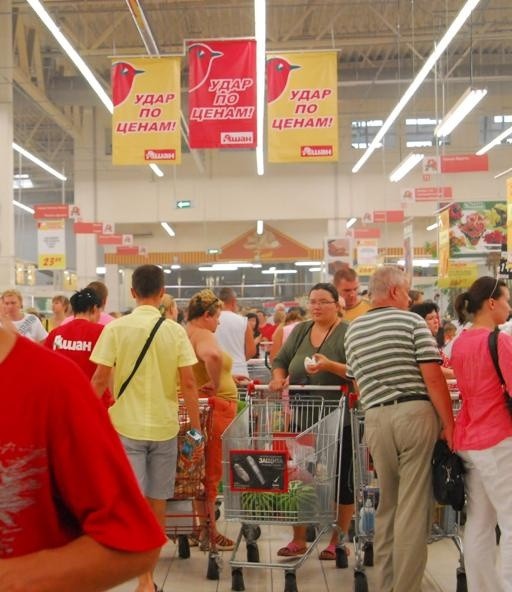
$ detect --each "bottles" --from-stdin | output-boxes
[358,498,377,542]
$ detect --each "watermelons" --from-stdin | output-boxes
[275,479,319,523]
[240,492,278,521]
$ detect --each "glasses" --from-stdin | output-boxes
[309,298,336,306]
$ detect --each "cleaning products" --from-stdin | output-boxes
[358,500,376,536]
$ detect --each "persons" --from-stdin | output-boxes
[448,276,512,592]
[408,289,512,536]
[1,326,168,592]
[1,282,117,408]
[89,263,205,592]
[348,264,458,592]
[160,269,374,559]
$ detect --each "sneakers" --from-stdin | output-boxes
[198,525,236,553]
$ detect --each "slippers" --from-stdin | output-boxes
[277,542,307,558]
[320,545,350,560]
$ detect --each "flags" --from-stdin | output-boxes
[107,55,181,166]
[264,48,341,162]
[183,38,259,149]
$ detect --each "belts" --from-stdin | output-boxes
[371,392,431,407]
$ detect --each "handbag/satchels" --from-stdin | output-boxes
[431,440,465,511]
